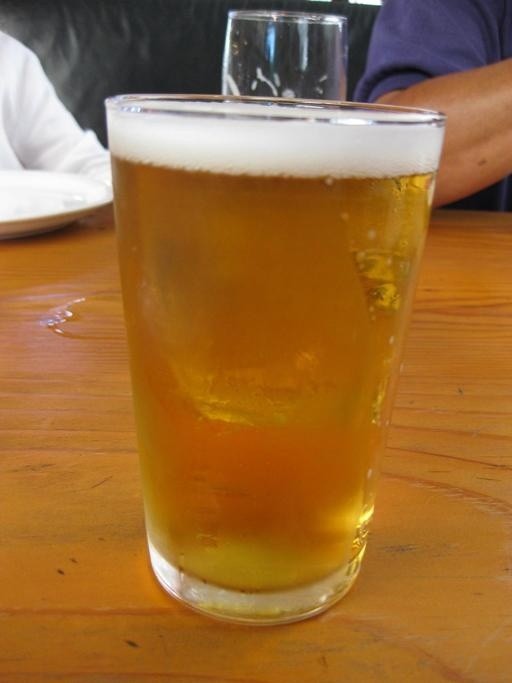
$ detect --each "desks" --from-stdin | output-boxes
[0,207,512,683]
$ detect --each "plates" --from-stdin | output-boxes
[0,169,112,238]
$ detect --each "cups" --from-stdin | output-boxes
[105,95,447,623]
[222,12,346,107]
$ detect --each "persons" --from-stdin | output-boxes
[352,0,511,212]
[0,31,111,188]
